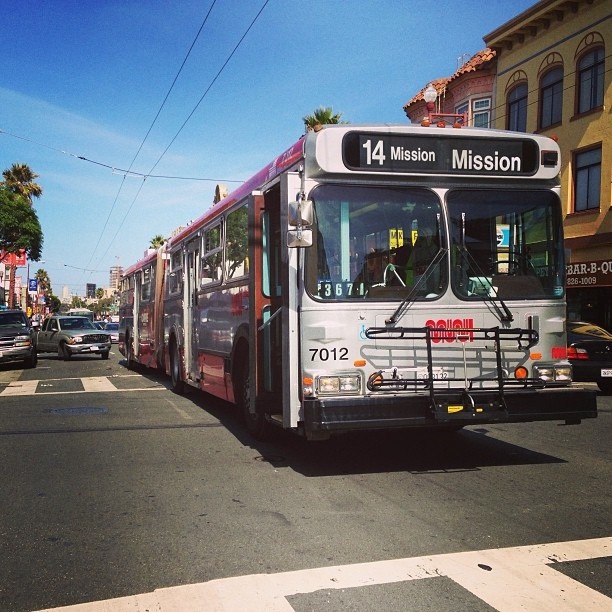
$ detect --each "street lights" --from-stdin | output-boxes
[25,261,45,314]
[424,82,467,127]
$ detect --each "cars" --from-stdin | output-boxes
[103,323,120,343]
[569,322,610,391]
[37,316,111,361]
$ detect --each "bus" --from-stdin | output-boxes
[118,120,598,443]
[66,308,94,324]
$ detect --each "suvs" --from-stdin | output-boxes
[0,311,37,368]
